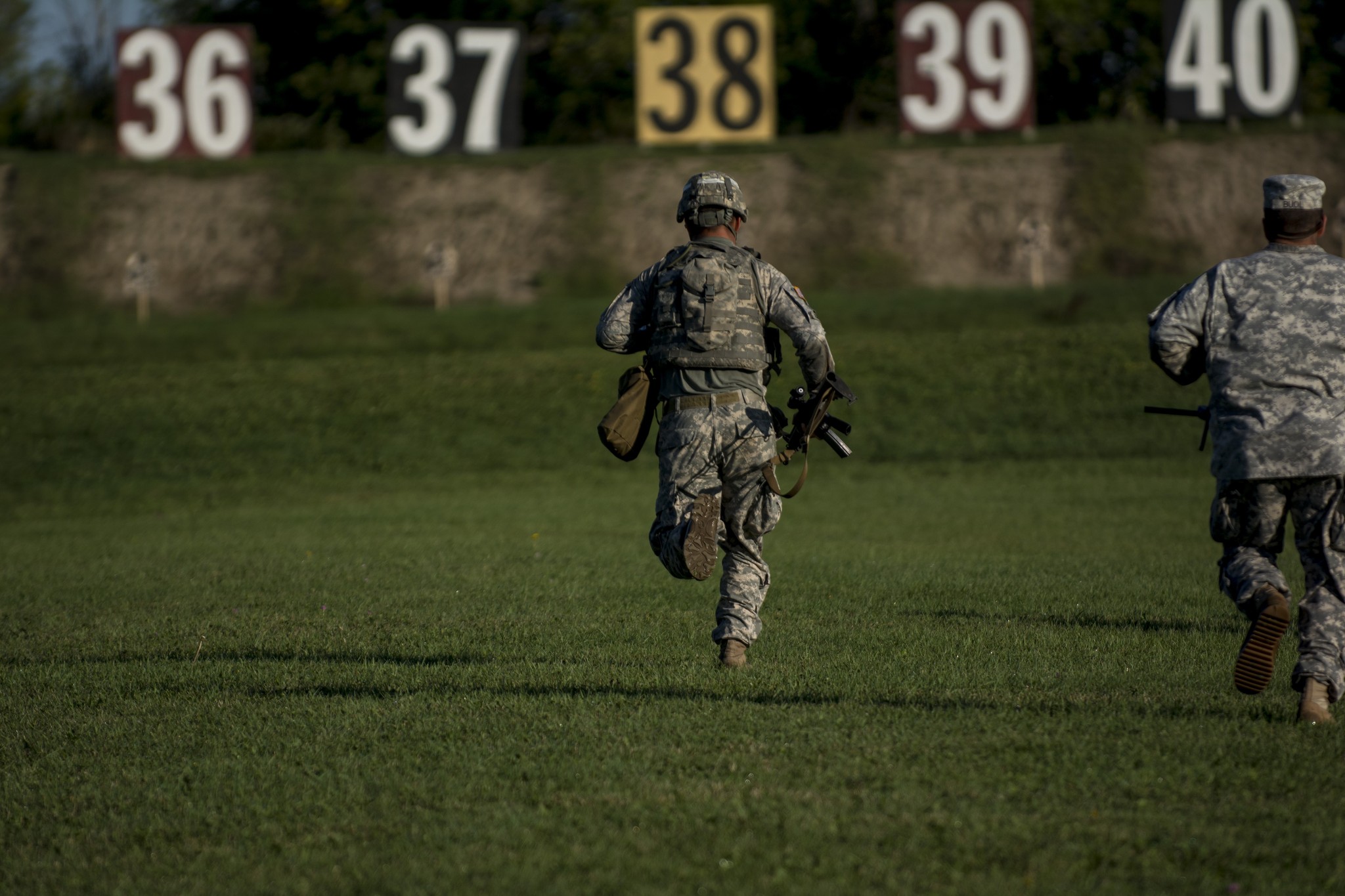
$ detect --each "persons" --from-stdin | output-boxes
[599,171,834,663]
[1148,174,1345,725]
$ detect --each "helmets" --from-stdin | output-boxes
[676,171,748,224]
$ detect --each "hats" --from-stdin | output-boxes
[1261,174,1326,210]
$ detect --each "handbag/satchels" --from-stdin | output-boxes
[598,362,660,462]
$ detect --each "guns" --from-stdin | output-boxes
[1141,393,1214,453]
[762,371,858,458]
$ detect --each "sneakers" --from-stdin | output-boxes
[720,638,754,671]
[1232,588,1291,695]
[1292,677,1337,726]
[680,493,721,581]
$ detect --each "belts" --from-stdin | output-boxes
[664,388,764,415]
